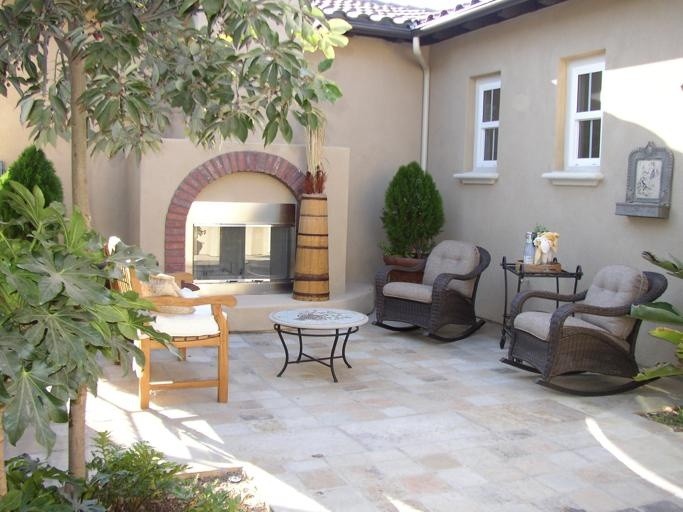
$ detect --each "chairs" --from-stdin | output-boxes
[501,264,668,397]
[103,242,237,411]
[373,238,492,342]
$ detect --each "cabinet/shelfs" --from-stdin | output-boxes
[500,254,584,351]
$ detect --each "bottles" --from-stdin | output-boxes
[523,232,534,264]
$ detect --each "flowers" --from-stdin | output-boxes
[533,230,559,264]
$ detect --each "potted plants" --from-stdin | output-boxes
[379,159,447,284]
